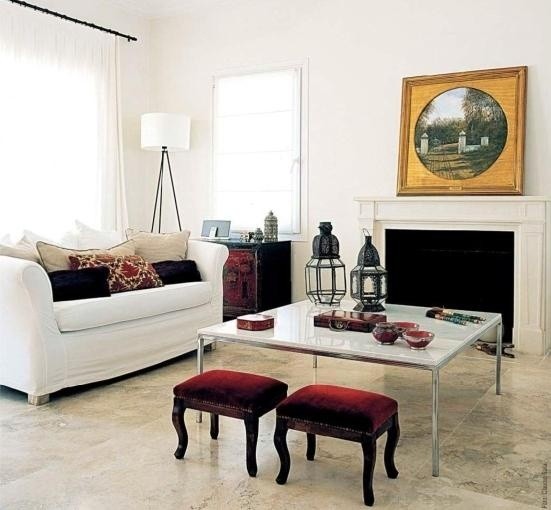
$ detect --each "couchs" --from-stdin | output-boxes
[0,239,229,405]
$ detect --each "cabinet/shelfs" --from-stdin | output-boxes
[203,240,292,318]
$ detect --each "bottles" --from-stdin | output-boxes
[372,322,398,345]
[253,227,264,242]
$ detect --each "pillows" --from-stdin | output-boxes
[126,228,190,263]
[0,236,42,266]
[152,259,202,285]
[37,241,135,272]
[68,254,164,292]
[47,266,111,302]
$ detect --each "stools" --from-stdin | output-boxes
[171,370,289,477]
[273,384,400,506]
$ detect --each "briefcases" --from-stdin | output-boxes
[314,310,386,333]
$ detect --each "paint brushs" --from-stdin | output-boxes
[426,307,486,325]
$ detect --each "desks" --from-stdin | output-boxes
[195,294,503,476]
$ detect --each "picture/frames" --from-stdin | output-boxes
[395,65,528,196]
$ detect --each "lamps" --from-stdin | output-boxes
[140,113,192,233]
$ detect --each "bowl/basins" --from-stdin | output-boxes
[394,322,435,350]
